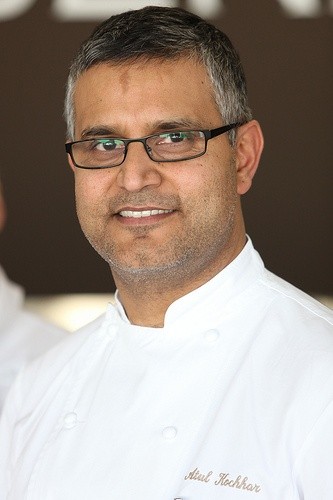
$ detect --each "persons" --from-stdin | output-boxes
[0,5,333,499]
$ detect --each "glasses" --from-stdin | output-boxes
[64,121,246,170]
